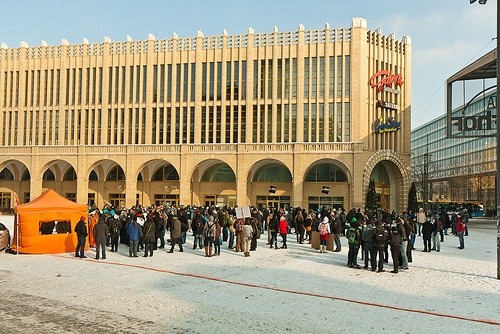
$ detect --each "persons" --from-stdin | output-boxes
[88,203,469,273]
[39,221,55,235]
[486,208,496,216]
[56,221,70,233]
[75,216,88,258]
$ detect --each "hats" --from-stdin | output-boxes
[80,216,86,220]
[351,217,357,222]
[194,210,201,213]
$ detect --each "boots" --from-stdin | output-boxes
[205,247,208,257]
[209,247,212,257]
[324,245,327,253]
[320,245,323,253]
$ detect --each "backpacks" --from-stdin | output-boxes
[348,228,359,244]
[375,228,385,244]
[319,222,327,235]
[365,227,374,243]
[205,222,215,238]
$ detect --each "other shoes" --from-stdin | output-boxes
[378,269,385,272]
[212,253,217,256]
[244,252,250,257]
[384,259,388,263]
[333,250,339,252]
[179,250,183,252]
[150,255,152,256]
[115,250,118,252]
[75,255,80,257]
[391,271,398,273]
[218,253,220,255]
[143,255,147,256]
[80,256,87,258]
[111,249,113,251]
[134,256,138,257]
[167,251,173,253]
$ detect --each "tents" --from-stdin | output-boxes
[10,188,90,255]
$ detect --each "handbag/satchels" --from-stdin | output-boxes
[181,223,189,232]
[255,223,260,238]
[248,229,253,239]
[306,226,311,231]
[287,226,290,230]
[110,223,118,233]
